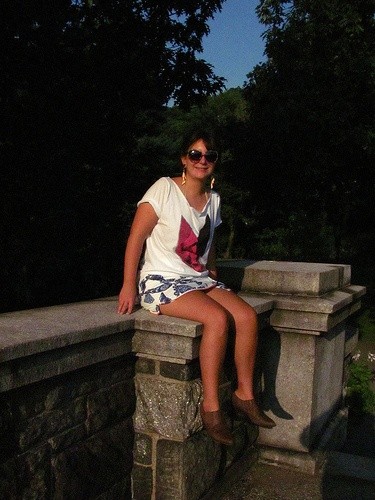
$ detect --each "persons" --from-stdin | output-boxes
[116,128,276,446]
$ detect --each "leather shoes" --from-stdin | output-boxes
[199,400,234,446]
[230,391,277,428]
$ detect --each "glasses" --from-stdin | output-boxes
[184,149,219,165]
